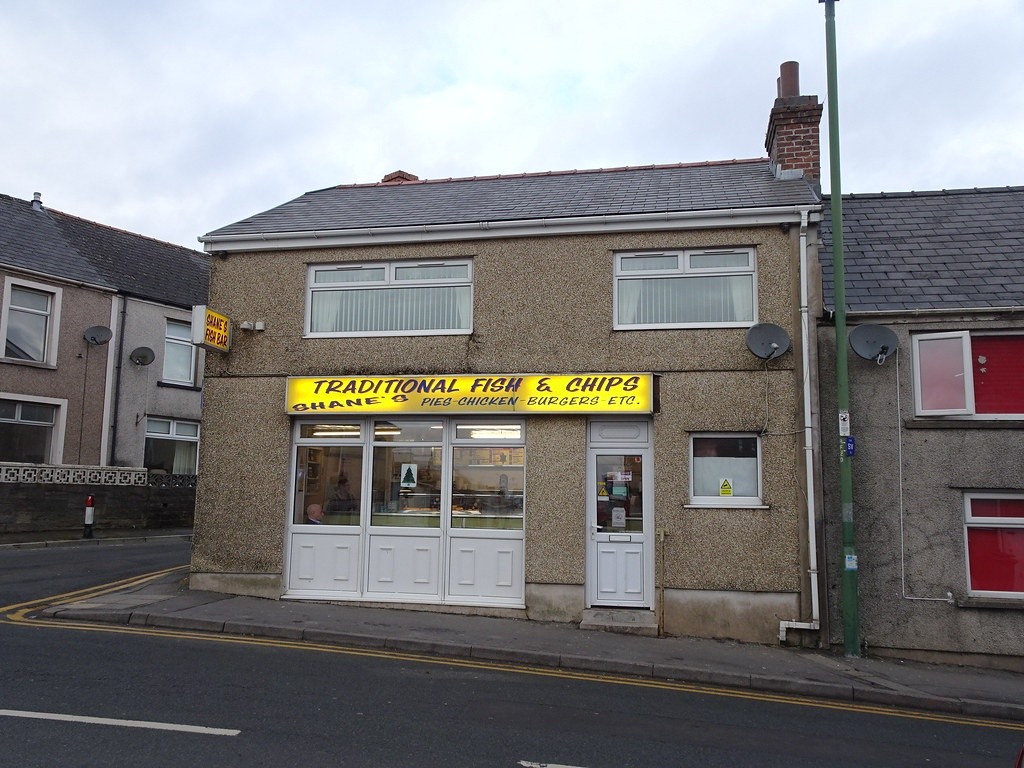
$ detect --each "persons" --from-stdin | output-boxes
[323,479,358,525]
[498,486,513,529]
[604,474,630,532]
[459,482,480,510]
[431,480,441,507]
[307,504,324,524]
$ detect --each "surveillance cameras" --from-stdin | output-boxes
[240,324,252,332]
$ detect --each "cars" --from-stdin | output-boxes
[597,492,629,532]
